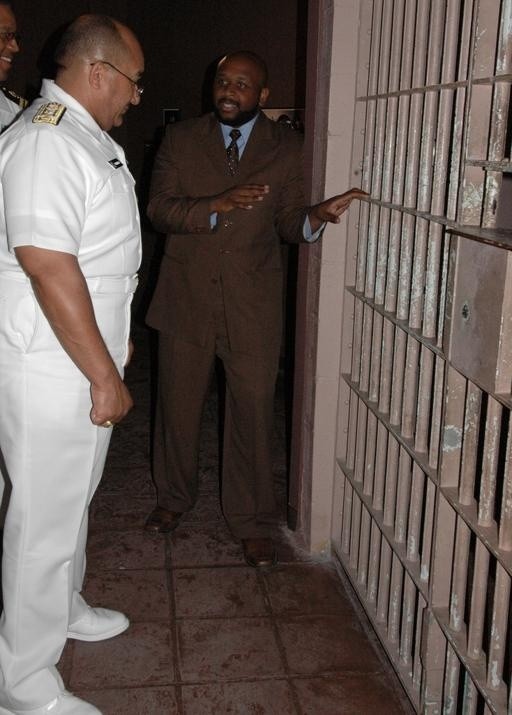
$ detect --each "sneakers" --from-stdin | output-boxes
[1,689,101,715]
[68,593,129,643]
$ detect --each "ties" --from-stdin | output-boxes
[225,130,243,177]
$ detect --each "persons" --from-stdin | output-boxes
[1,13,144,714]
[146,49,370,567]
[1,1,30,132]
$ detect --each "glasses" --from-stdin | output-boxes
[91,60,144,96]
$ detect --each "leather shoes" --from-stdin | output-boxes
[145,506,183,532]
[241,536,277,566]
